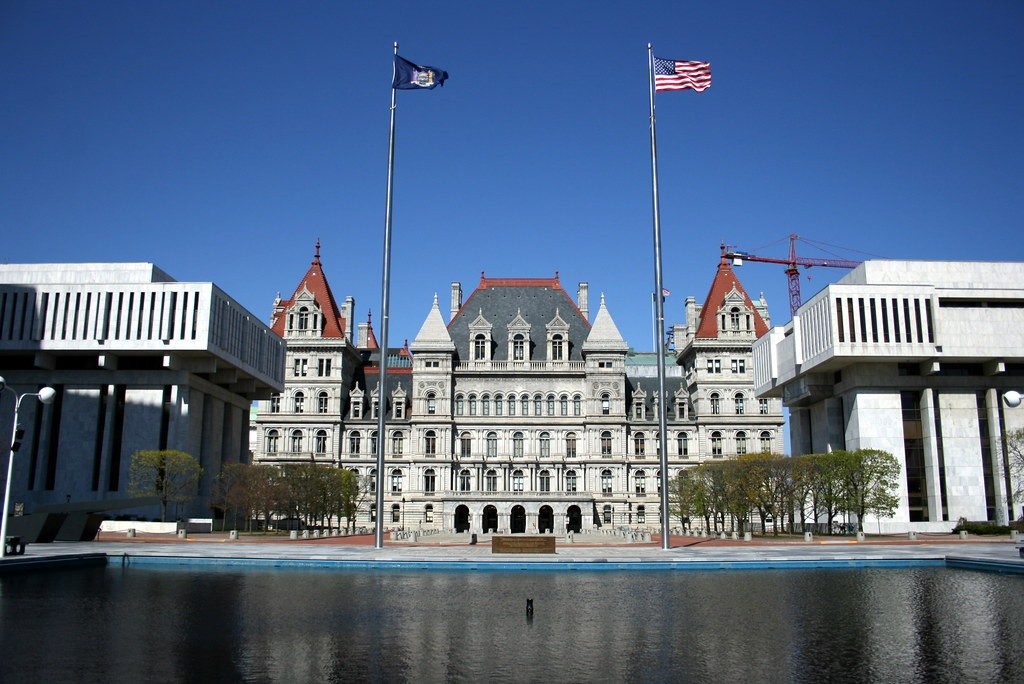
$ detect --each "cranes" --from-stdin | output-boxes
[720,232,891,321]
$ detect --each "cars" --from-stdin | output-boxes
[260,517,308,533]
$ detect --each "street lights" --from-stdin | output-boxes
[0,376,57,556]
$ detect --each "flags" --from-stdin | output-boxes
[652,56,712,94]
[391,53,449,91]
